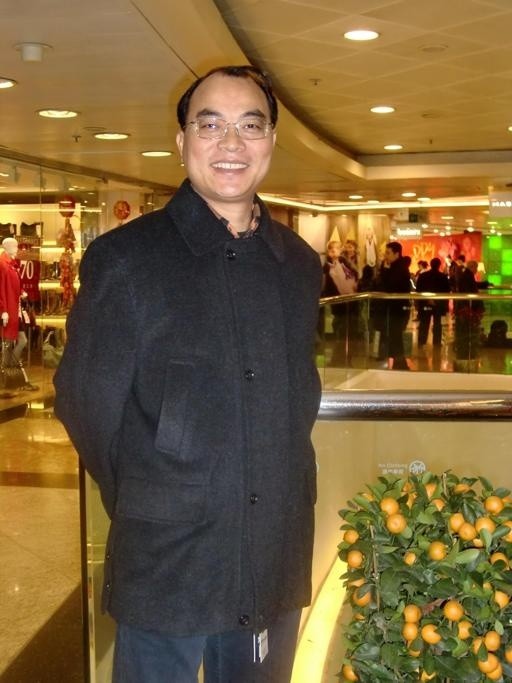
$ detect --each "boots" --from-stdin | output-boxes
[40,289,75,315]
[40,261,60,279]
[29,325,41,353]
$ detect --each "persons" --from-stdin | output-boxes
[323,236,496,371]
[364,226,378,277]
[0,234,39,393]
[51,63,326,683]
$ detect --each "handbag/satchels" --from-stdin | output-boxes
[0,223,37,237]
[43,331,64,370]
[2,366,26,388]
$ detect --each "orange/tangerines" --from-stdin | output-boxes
[341,480,512,683]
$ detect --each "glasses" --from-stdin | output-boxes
[182,121,273,139]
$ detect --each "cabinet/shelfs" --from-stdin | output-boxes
[0,192,100,390]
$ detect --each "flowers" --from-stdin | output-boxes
[453,306,479,359]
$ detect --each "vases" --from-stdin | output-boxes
[454,360,480,375]
